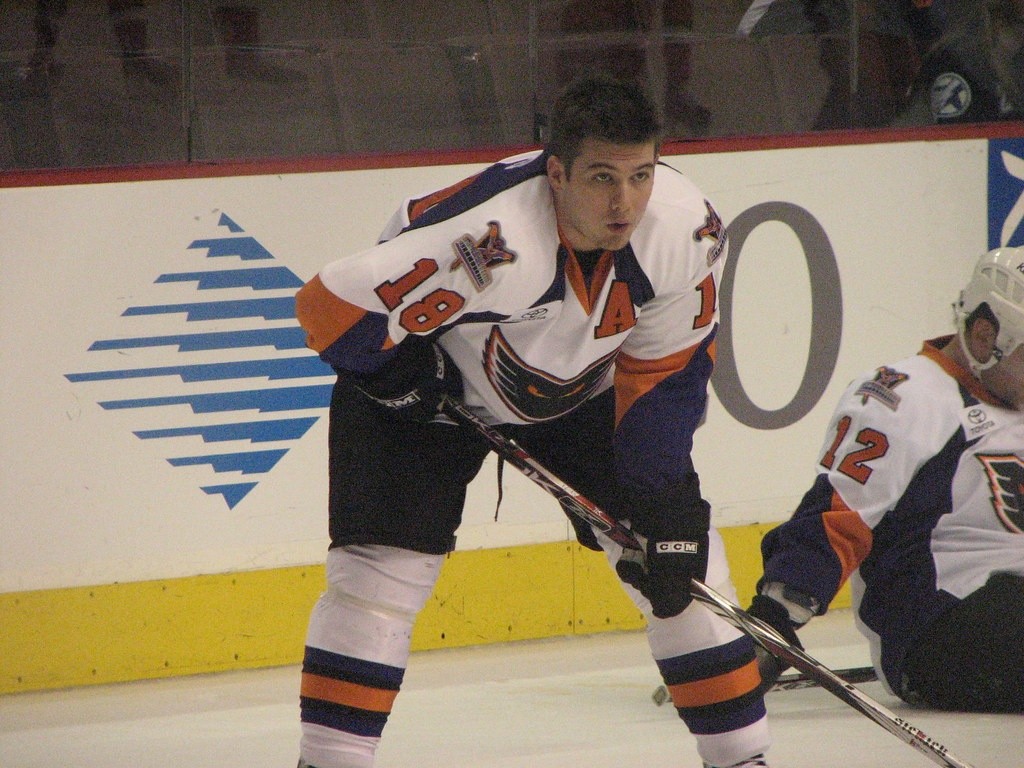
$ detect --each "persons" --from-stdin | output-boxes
[292,83,774,768]
[29,0,307,84]
[552,0,1024,139]
[745,246,1024,716]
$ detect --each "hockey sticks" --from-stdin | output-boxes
[650,666,878,708]
[441,394,979,768]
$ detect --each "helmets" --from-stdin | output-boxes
[955,248,1024,346]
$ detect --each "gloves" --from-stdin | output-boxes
[345,334,466,425]
[616,499,710,619]
[741,595,805,696]
